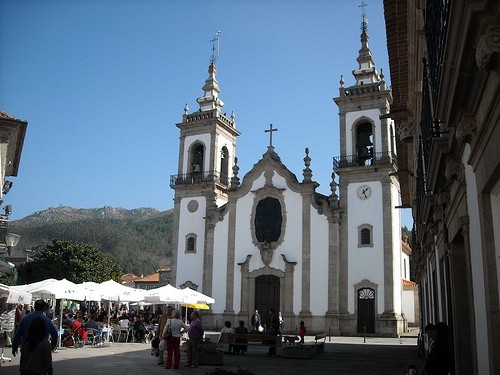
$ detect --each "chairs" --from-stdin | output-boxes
[86,324,131,346]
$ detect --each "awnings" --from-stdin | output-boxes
[4,277,217,314]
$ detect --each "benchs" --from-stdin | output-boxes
[221,333,276,354]
[311,333,327,353]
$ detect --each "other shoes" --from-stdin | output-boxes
[157,360,164,364]
[189,364,197,368]
[184,363,191,366]
[156,353,159,357]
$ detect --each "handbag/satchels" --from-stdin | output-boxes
[163,328,172,340]
[159,339,167,351]
[152,337,160,349]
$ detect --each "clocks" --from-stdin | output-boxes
[357,185,372,200]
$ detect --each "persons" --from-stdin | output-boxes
[217,320,235,350]
[251,310,260,331]
[151,305,174,365]
[6,302,191,348]
[264,308,280,358]
[420,321,451,375]
[163,309,190,369]
[186,309,205,367]
[298,321,306,344]
[11,299,58,375]
[231,320,248,352]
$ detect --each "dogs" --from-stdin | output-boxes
[282,336,299,345]
